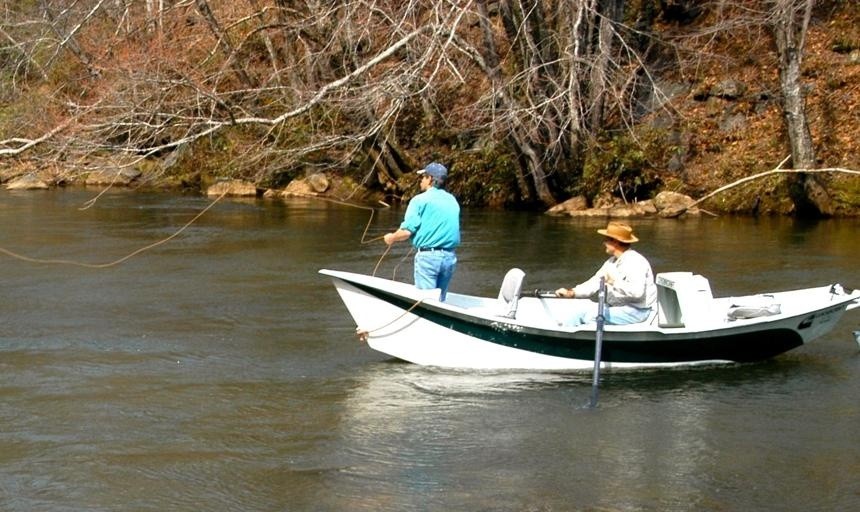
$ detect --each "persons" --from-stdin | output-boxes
[383,162,461,302]
[555,221,658,327]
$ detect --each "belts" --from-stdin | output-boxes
[420,247,452,251]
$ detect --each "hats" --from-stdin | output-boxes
[597,221,640,244]
[416,162,448,178]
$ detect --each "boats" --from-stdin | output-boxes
[319,268,860,370]
